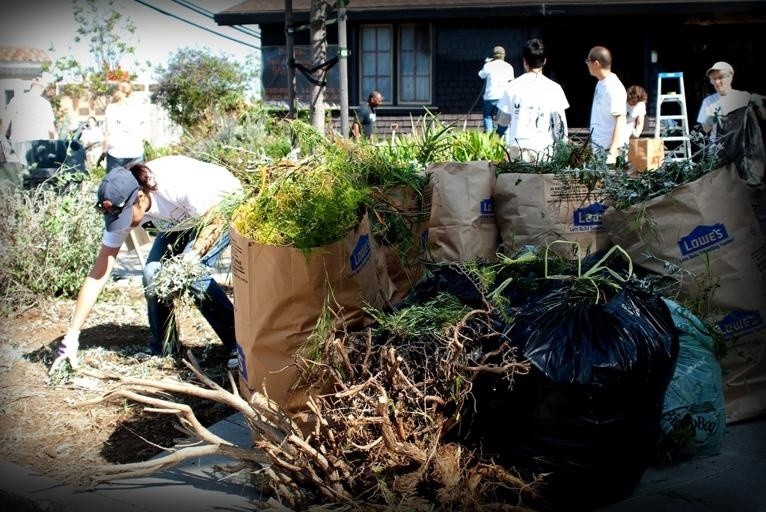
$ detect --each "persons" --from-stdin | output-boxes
[79,115,105,162]
[88,79,152,176]
[625,84,648,144]
[479,46,516,138]
[696,61,750,162]
[350,90,382,144]
[585,46,629,164]
[48,156,257,370]
[0,76,59,165]
[494,38,571,164]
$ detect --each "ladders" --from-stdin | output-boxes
[655,71,693,171]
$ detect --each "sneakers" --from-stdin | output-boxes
[142,342,156,355]
[228,349,241,366]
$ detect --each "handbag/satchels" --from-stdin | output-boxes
[629,136,664,178]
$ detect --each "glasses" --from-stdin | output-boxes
[94,186,137,215]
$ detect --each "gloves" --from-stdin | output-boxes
[48,329,81,376]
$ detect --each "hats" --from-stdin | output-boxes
[494,45,505,55]
[94,164,142,232]
[707,60,733,74]
